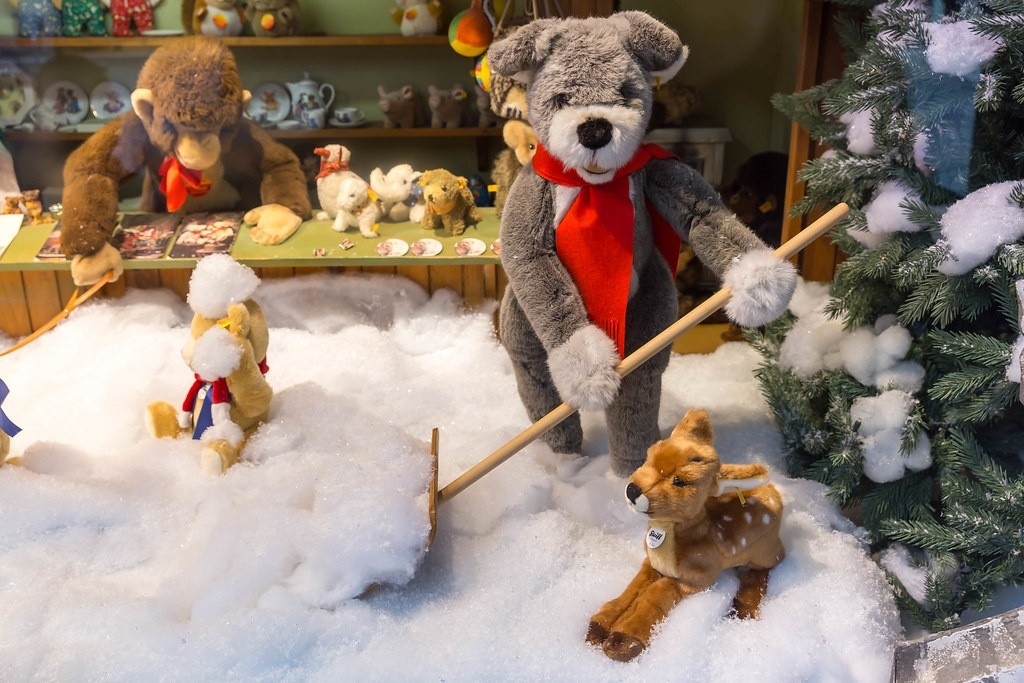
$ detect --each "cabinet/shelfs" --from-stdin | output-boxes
[0,0,504,207]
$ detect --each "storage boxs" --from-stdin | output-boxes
[642,119,734,187]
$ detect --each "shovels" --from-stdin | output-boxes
[355,202,850,603]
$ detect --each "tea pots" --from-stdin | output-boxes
[285,72,334,129]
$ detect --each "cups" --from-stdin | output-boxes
[335,108,365,122]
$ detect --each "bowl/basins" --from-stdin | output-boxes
[277,120,298,129]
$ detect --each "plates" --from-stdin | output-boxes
[492,237,501,255]
[140,28,184,37]
[330,118,366,127]
[41,79,89,125]
[455,237,486,256]
[377,237,409,256]
[244,83,290,129]
[89,81,134,120]
[410,238,443,256]
